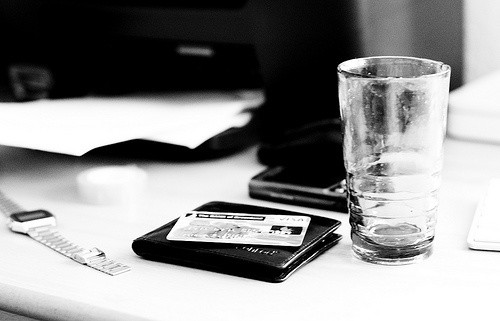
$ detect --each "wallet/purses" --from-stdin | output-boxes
[129,200,342,285]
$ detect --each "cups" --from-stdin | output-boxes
[337,56,451,266]
